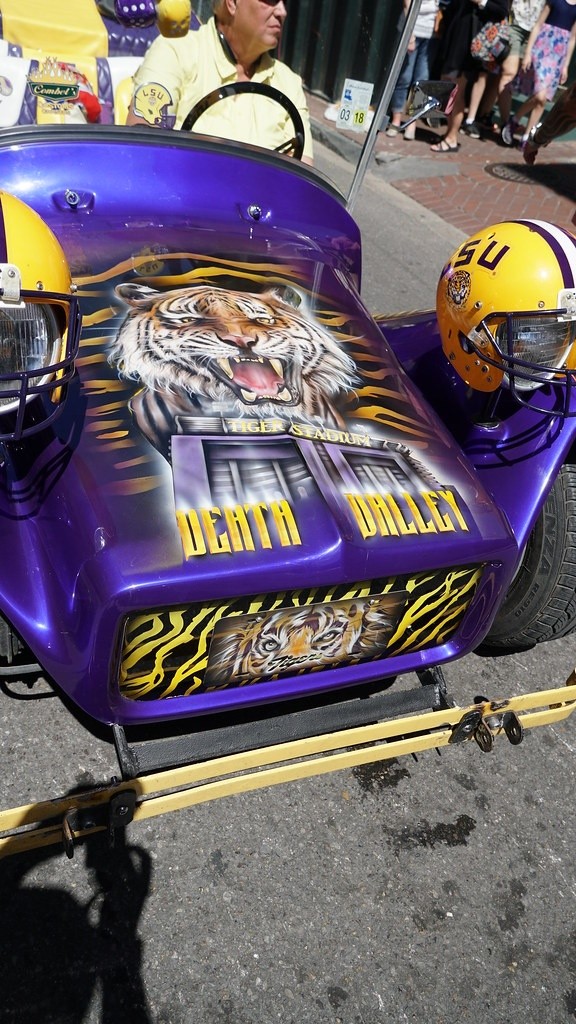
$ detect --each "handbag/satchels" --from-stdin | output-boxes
[471,12,512,70]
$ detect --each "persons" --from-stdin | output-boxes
[125,0,315,168]
[394,0,576,174]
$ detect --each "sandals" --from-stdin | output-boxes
[428,135,457,153]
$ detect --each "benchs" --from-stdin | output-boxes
[0,0,206,128]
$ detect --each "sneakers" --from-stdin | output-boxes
[460,120,481,139]
[502,115,518,145]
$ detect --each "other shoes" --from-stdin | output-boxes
[387,126,415,140]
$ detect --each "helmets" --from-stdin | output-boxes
[436,217,576,418]
